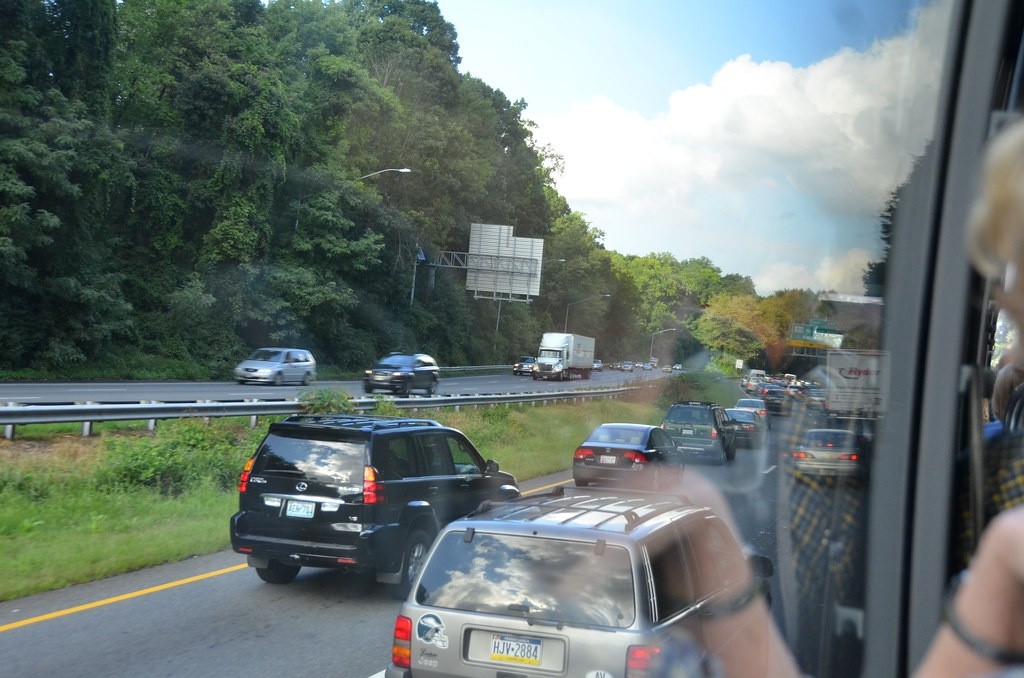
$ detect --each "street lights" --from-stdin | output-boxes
[493,258,566,351]
[649,328,676,359]
[295,169,411,232]
[565,294,611,335]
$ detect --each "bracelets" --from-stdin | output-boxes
[702,580,764,619]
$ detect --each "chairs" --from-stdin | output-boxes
[631,437,641,445]
[599,435,609,443]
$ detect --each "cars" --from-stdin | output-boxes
[734,368,828,429]
[573,423,686,496]
[592,360,604,371]
[725,407,767,448]
[513,355,536,375]
[663,364,672,373]
[610,361,634,373]
[673,363,683,370]
[794,428,873,482]
[636,359,660,370]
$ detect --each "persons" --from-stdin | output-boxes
[627,118,1024,678]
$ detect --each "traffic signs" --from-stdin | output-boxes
[791,325,844,350]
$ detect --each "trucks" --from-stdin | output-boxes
[530,332,596,381]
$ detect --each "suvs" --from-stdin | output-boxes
[385,484,776,678]
[363,352,440,397]
[230,413,521,601]
[658,400,738,465]
[234,348,318,385]
